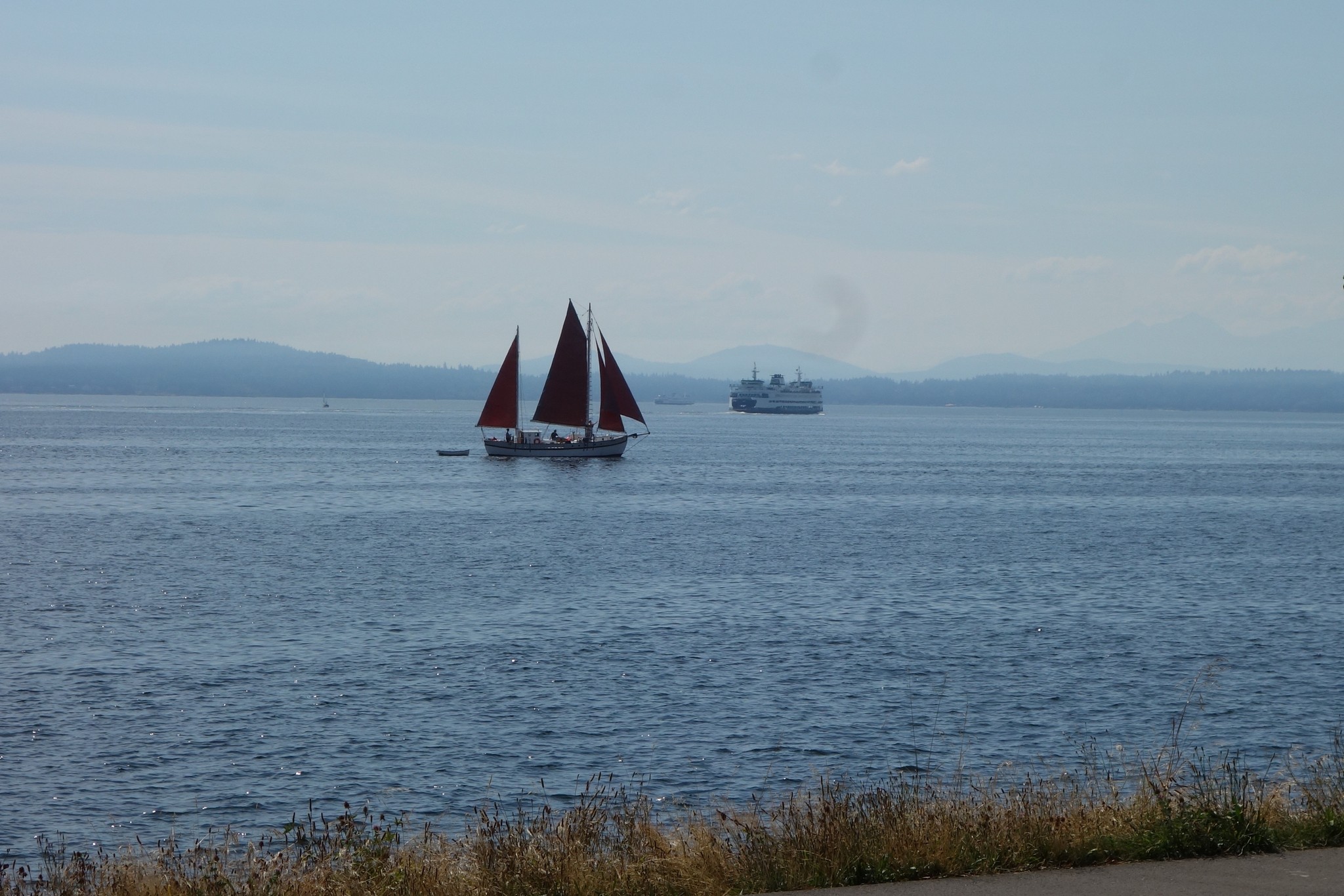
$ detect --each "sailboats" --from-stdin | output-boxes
[475,298,651,457]
[322,394,330,409]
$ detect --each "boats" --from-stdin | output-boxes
[654,393,695,405]
[436,449,470,456]
[729,362,824,414]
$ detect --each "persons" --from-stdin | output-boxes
[589,420,598,438]
[506,429,511,442]
[549,429,558,440]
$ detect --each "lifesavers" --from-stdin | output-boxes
[534,438,540,444]
